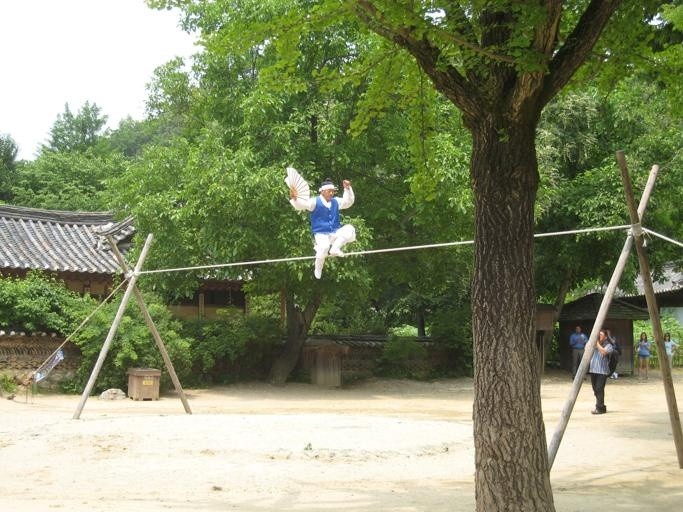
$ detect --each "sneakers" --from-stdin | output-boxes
[328,248,345,258]
[314,263,323,279]
[591,405,606,415]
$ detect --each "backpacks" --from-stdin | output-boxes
[602,342,619,377]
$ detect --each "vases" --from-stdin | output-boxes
[127,367,162,401]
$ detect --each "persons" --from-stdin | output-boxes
[605,328,618,379]
[663,332,678,373]
[635,332,650,380]
[568,325,588,382]
[289,178,356,281]
[588,329,613,414]
